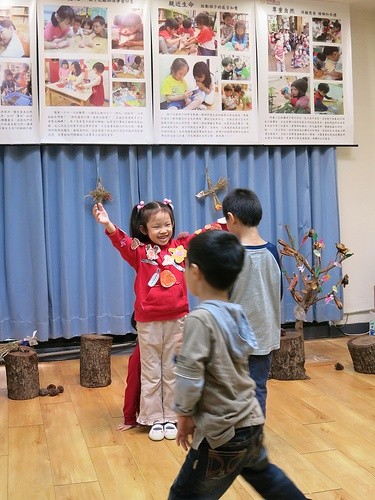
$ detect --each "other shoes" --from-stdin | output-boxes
[164,422,178,439]
[149,424,164,441]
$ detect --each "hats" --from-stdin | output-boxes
[291,77,308,100]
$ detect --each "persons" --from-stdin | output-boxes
[160,8,252,110]
[44,5,146,106]
[0,15,32,106]
[166,228,314,500]
[267,15,343,115]
[93,189,283,441]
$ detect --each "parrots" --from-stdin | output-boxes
[287,271,299,290]
